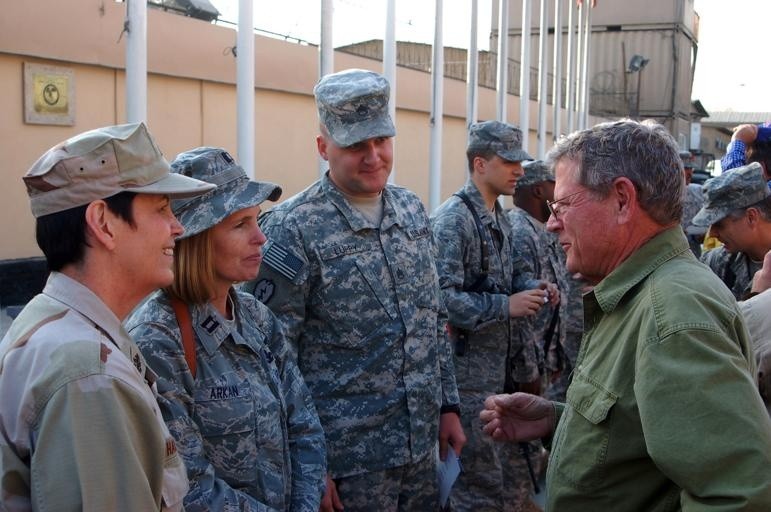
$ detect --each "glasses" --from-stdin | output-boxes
[547,178,616,222]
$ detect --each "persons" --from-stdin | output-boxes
[429,119,560,512]
[505,159,569,512]
[0,122,217,512]
[691,161,771,298]
[122,146,327,512]
[737,249,770,416]
[721,123,771,189]
[477,117,771,512]
[678,150,705,262]
[236,68,466,512]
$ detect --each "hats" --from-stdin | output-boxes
[691,162,770,226]
[512,159,556,188]
[466,121,535,161]
[679,151,697,169]
[167,147,282,241]
[314,69,395,148]
[23,122,217,218]
